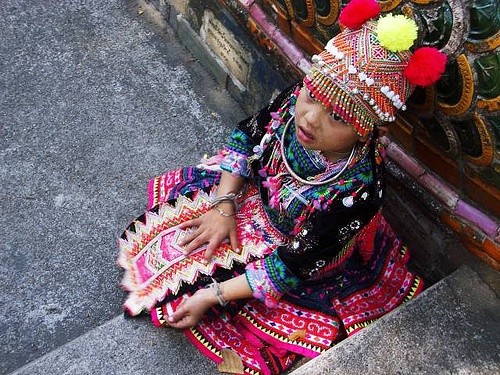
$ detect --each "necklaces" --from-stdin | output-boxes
[280,113,358,186]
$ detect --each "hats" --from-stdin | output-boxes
[302,0,447,139]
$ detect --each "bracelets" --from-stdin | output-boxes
[207,193,241,218]
[208,281,231,307]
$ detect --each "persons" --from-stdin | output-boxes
[114,1,447,375]
